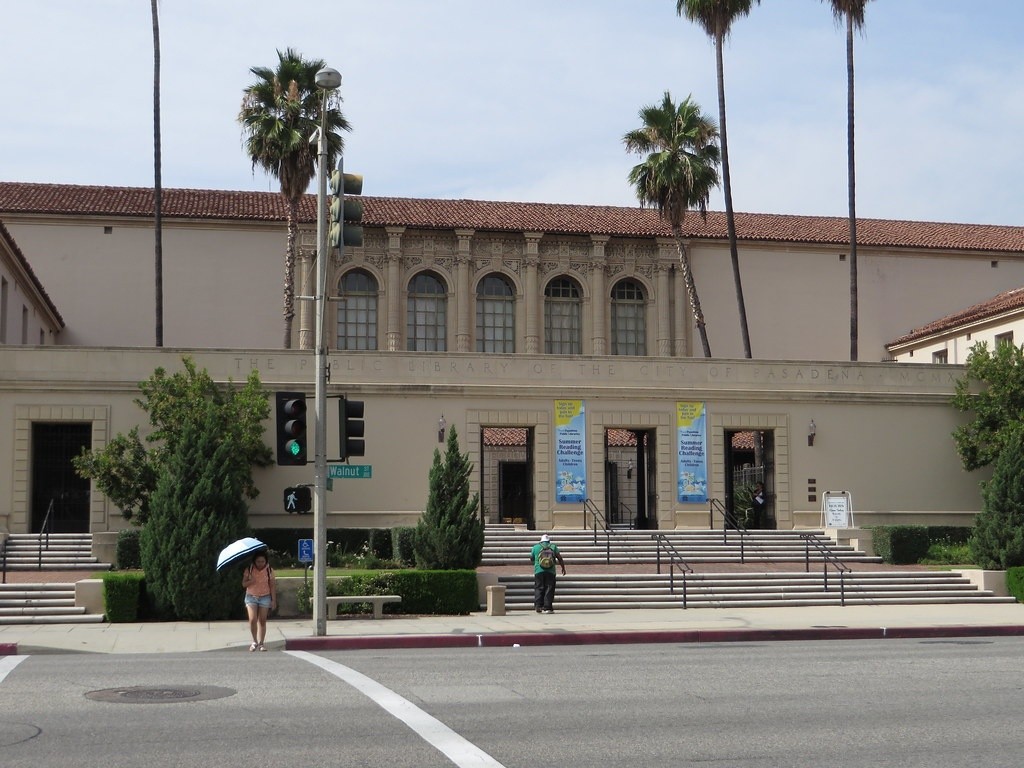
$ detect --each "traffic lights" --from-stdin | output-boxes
[284,487,312,512]
[276,392,307,466]
[337,400,365,456]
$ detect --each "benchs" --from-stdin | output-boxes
[308,595,402,620]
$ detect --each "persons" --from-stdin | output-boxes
[751,481,765,528]
[531,534,566,614]
[242,552,276,651]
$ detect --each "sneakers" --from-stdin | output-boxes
[258,645,266,652]
[545,610,554,614]
[535,604,542,613]
[250,642,258,652]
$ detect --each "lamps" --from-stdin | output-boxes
[807,420,817,447]
[437,411,446,443]
[627,458,633,479]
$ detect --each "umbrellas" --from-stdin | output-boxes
[216,536,268,578]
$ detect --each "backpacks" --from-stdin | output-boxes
[538,543,556,569]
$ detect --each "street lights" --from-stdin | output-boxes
[313,66,341,636]
[329,172,364,248]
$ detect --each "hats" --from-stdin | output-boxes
[540,534,550,542]
